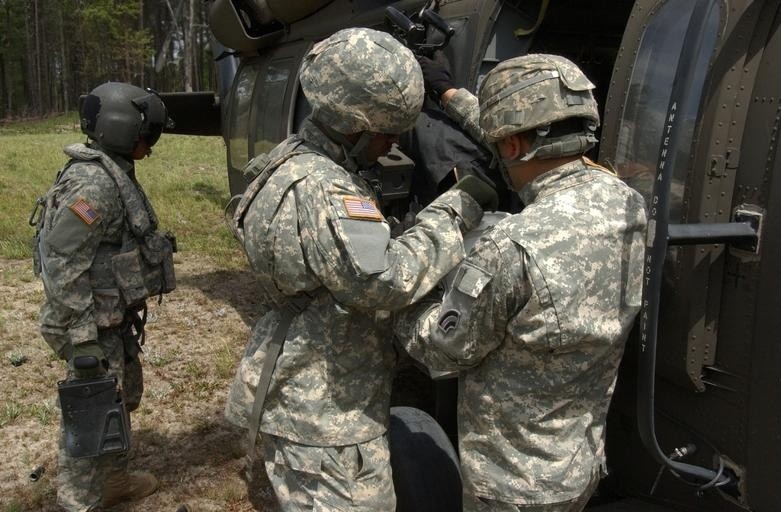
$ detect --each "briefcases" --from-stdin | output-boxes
[57,374,129,458]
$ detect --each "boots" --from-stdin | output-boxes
[100,447,158,509]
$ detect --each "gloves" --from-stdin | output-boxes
[73,344,109,378]
[449,176,499,213]
[414,51,456,110]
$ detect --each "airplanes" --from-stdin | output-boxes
[155,0,781,512]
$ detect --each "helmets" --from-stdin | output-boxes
[479,53,600,143]
[299,28,425,133]
[77,83,165,153]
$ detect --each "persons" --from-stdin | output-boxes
[32,82,177,512]
[393,50,648,512]
[225,27,498,512]
